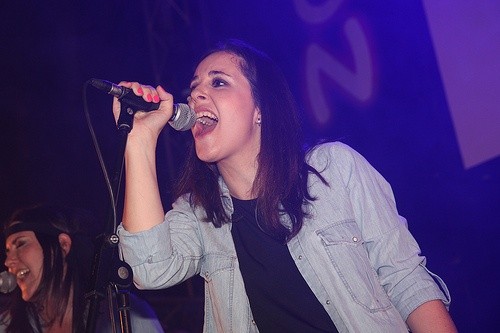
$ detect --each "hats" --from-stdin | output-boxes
[1,204,70,241]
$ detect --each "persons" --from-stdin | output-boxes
[111,41,460,333]
[0,203,165,333]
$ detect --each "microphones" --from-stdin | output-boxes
[90,78,196,134]
[0,270,17,294]
